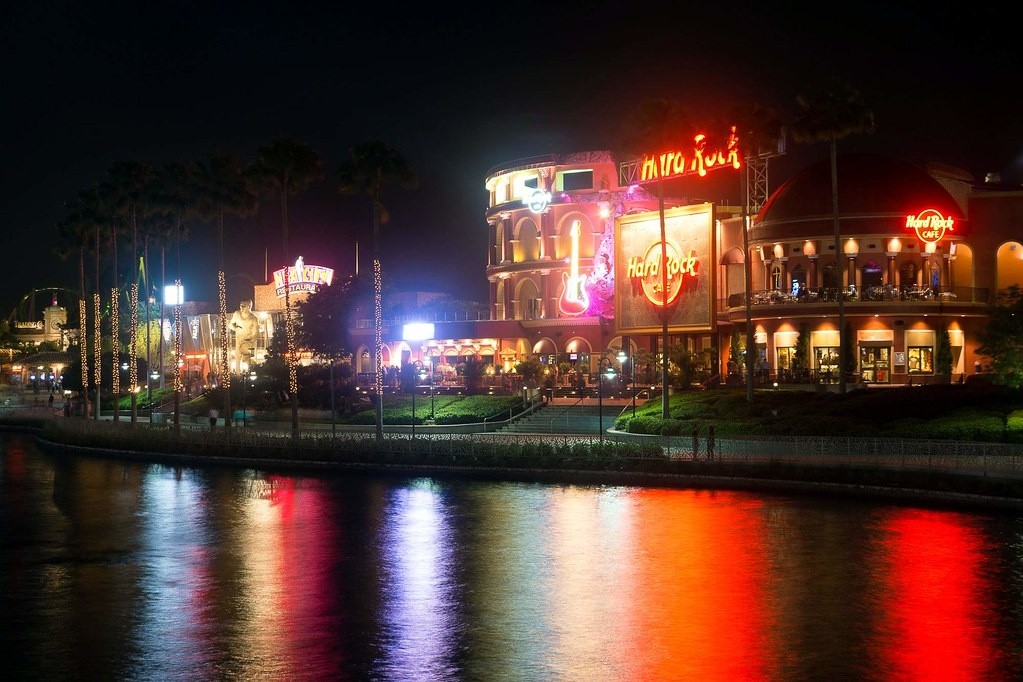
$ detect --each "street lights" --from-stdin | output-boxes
[243,366,258,427]
[599,357,617,443]
[149,363,160,423]
[411,360,428,438]
[616,343,636,418]
[422,348,434,420]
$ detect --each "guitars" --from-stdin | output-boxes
[558,220,590,315]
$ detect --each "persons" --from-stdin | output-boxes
[208,405,218,431]
[726,358,733,375]
[48,394,54,407]
[382,361,464,387]
[751,281,940,305]
[229,298,259,375]
[762,358,770,382]
[706,426,715,461]
[63,398,72,417]
[692,423,699,461]
[545,376,553,404]
